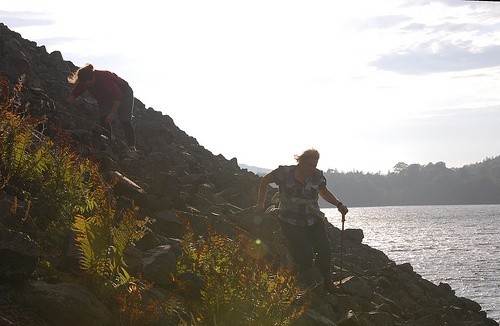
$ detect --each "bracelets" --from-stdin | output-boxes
[337,202,342,208]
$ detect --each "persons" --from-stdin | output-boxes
[255,148,349,283]
[67,64,134,152]
